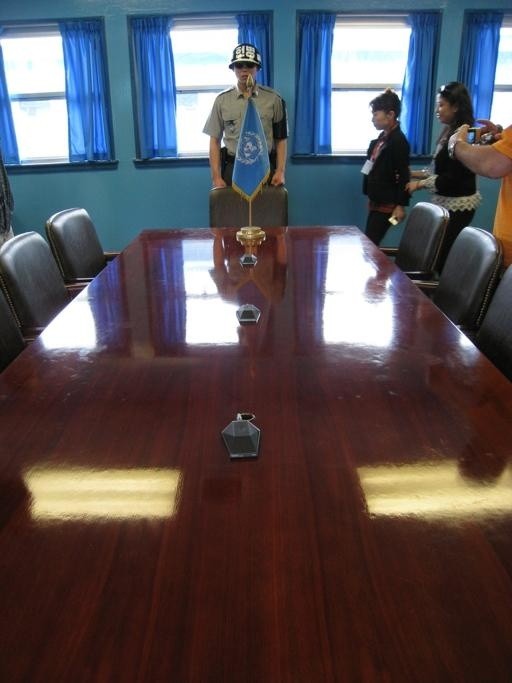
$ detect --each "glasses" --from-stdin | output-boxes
[440,85,447,94]
[234,62,256,68]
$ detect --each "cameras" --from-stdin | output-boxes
[466,128,481,144]
[480,132,494,145]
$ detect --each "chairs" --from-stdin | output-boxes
[0,286,35,372]
[0,231,90,334]
[44,206,120,282]
[412,226,503,340]
[473,262,512,382]
[378,201,451,279]
[208,183,289,229]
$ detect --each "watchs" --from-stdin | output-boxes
[448,139,468,158]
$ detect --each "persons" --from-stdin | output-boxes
[405,81,481,276]
[203,43,289,189]
[362,89,412,248]
[448,119,512,277]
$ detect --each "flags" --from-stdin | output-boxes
[232,97,270,201]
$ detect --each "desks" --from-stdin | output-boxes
[0,224,512,683]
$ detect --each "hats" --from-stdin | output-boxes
[229,43,262,71]
